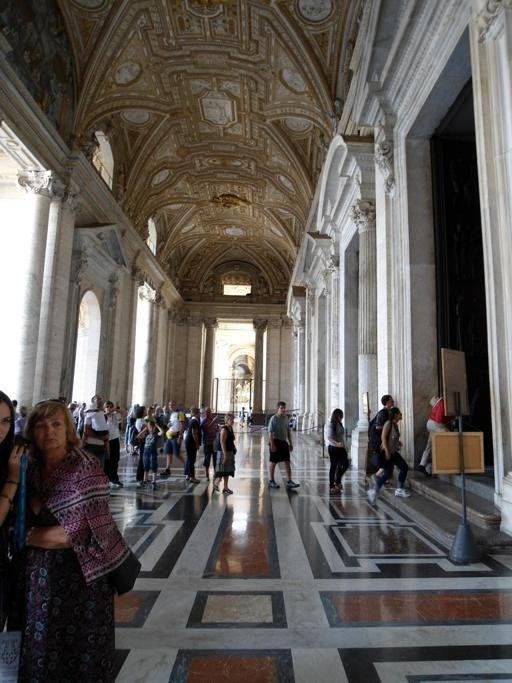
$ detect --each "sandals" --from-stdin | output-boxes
[223,488,233,494]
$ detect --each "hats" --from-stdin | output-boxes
[429,395,442,407]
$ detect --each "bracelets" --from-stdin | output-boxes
[4,479,21,485]
[0,493,13,507]
[24,524,35,547]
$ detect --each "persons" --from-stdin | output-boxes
[12,400,28,433]
[125,400,237,495]
[418,397,453,476]
[17,398,132,682]
[239,406,253,428]
[326,409,349,495]
[366,395,413,504]
[267,402,300,489]
[0,387,29,683]
[59,396,124,488]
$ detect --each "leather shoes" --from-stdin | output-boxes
[416,465,427,475]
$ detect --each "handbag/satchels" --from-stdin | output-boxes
[214,432,221,451]
[369,449,382,467]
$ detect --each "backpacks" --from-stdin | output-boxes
[368,408,388,445]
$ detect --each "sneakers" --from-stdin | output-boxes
[136,479,159,490]
[269,481,280,487]
[286,481,299,487]
[366,473,389,502]
[395,487,410,497]
[186,474,200,482]
[110,481,122,487]
[160,468,170,474]
